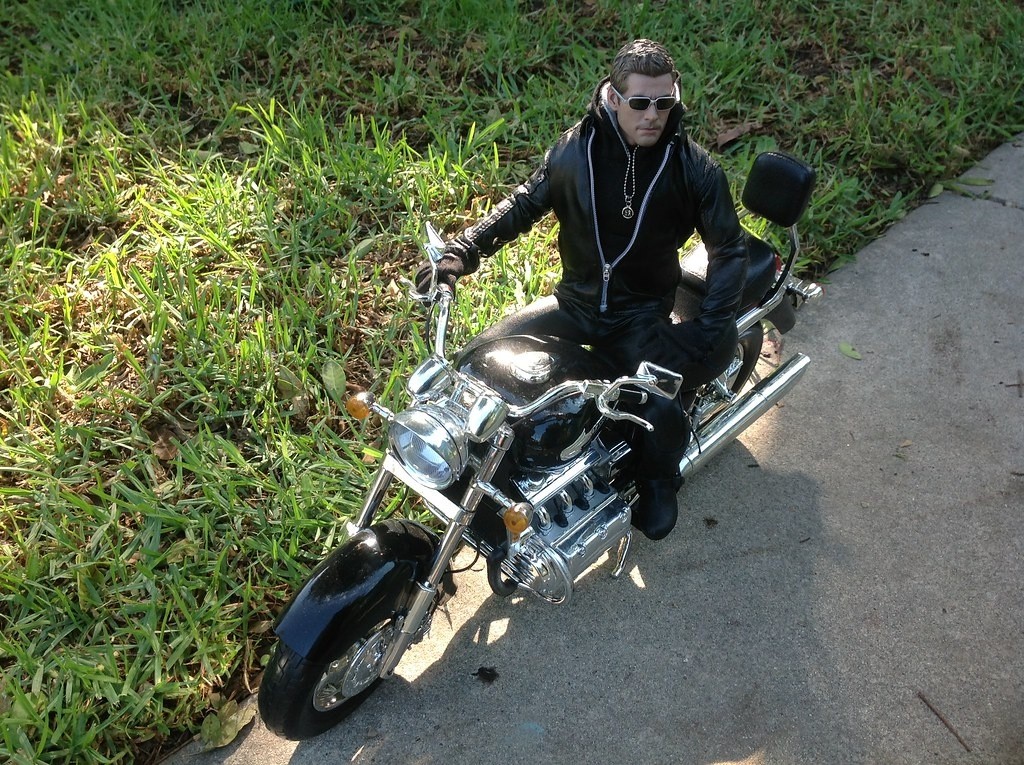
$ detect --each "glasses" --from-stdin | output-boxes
[610,85,676,112]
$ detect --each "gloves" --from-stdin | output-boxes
[637,320,707,376]
[415,235,480,308]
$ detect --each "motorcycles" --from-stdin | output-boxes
[258,147,824,740]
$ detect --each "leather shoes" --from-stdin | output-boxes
[639,464,685,540]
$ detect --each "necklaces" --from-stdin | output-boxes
[621,144,639,219]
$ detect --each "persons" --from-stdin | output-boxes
[412,39,750,542]
